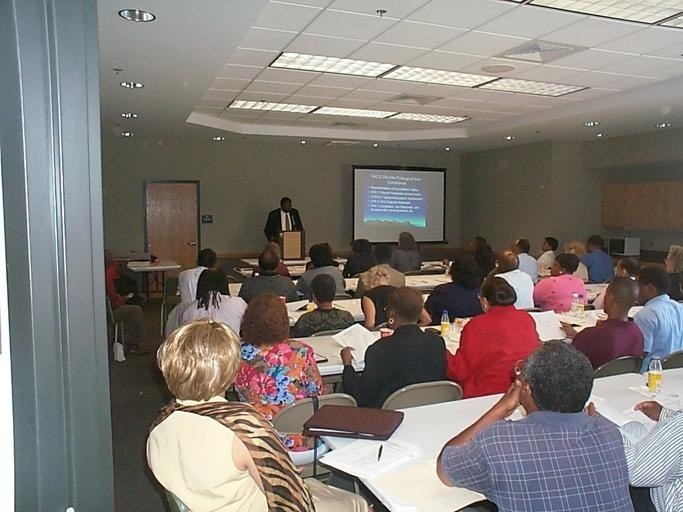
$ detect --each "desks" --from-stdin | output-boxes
[124,258,181,337]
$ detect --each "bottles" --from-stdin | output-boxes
[441,309,449,337]
[571,293,585,316]
[147,244,153,264]
[540,260,545,274]
[648,356,663,392]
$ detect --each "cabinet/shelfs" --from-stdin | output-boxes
[601,181,682,232]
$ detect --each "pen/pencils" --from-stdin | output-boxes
[378,445,383,461]
[560,321,581,327]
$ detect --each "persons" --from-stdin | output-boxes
[436,339,634,512]
[103,249,154,354]
[423,232,683,399]
[264,197,303,245]
[147,318,373,512]
[585,400,683,512]
[179,232,448,466]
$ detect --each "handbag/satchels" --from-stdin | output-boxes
[113,343,126,361]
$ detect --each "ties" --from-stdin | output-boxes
[286,213,290,231]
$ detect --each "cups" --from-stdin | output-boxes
[380,328,391,337]
